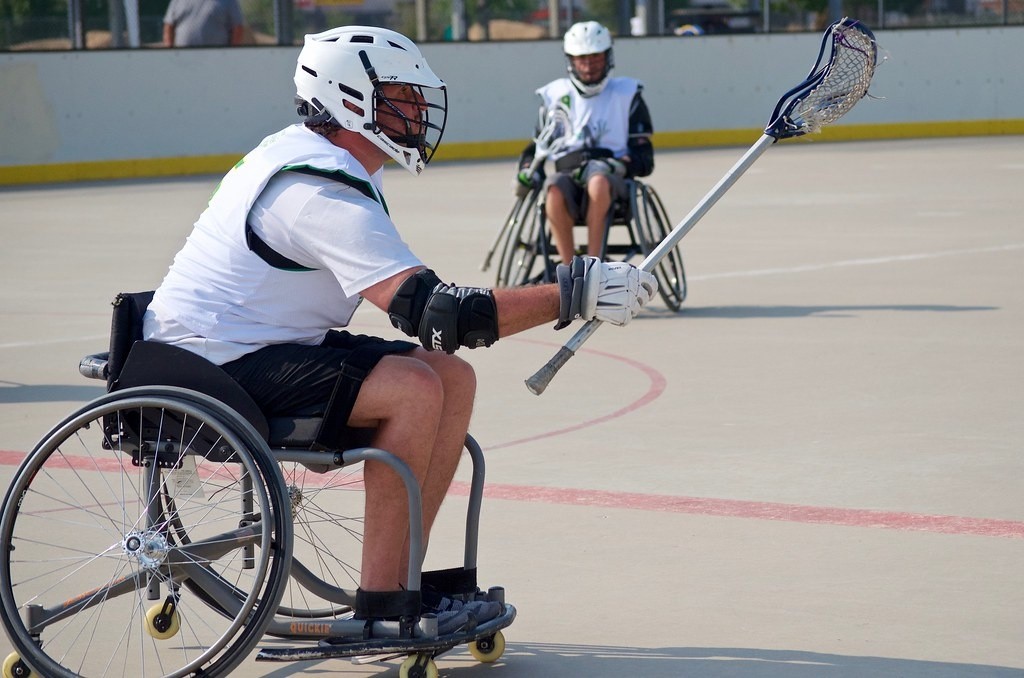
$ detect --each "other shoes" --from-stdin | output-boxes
[418,596,504,635]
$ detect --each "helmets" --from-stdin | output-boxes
[292,25,448,177]
[563,20,616,97]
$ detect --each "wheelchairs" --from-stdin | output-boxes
[0,291,517,678]
[497,181,687,313]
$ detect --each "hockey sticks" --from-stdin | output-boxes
[479,100,574,274]
[521,15,887,400]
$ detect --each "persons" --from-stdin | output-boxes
[143,26,657,635]
[539,20,656,266]
[164,0,256,49]
[829,0,885,28]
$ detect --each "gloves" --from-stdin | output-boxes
[580,157,627,188]
[512,168,539,201]
[553,255,659,332]
